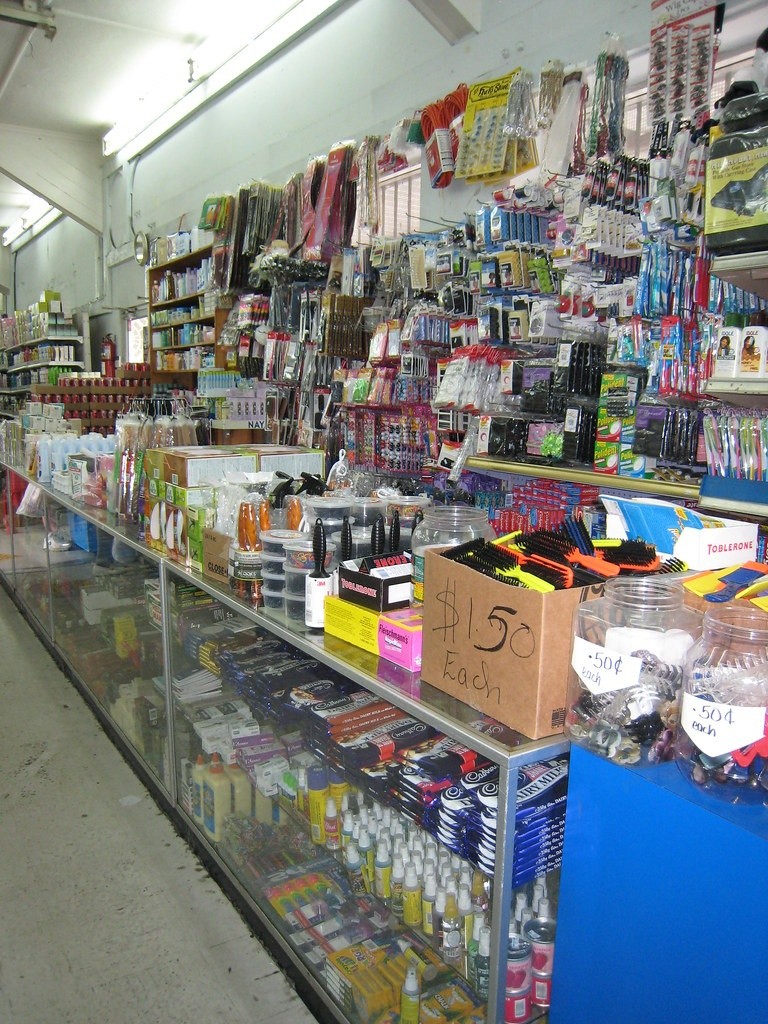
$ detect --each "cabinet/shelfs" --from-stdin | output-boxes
[0,458,571,1024]
[145,242,216,398]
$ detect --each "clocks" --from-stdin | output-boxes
[131,230,149,267]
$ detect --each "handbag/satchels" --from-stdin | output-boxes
[16,483,45,518]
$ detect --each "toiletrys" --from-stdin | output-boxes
[37,432,114,482]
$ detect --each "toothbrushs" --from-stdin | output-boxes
[634,232,768,481]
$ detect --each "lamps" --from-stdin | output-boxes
[100,0,305,158]
[2,199,54,247]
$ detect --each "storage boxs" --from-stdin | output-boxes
[325,927,488,1024]
[15,400,83,438]
[472,201,553,342]
[67,508,135,622]
[9,290,79,367]
[701,122,768,258]
[136,443,759,800]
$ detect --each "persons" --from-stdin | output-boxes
[718,336,730,356]
[741,336,755,360]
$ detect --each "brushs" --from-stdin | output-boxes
[440,510,683,598]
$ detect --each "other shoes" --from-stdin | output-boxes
[43,533,72,552]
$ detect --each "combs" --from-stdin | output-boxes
[703,567,764,603]
[439,512,687,593]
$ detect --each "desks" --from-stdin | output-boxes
[548,740,768,1024]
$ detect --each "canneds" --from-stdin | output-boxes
[503,916,556,1024]
[32,362,149,437]
